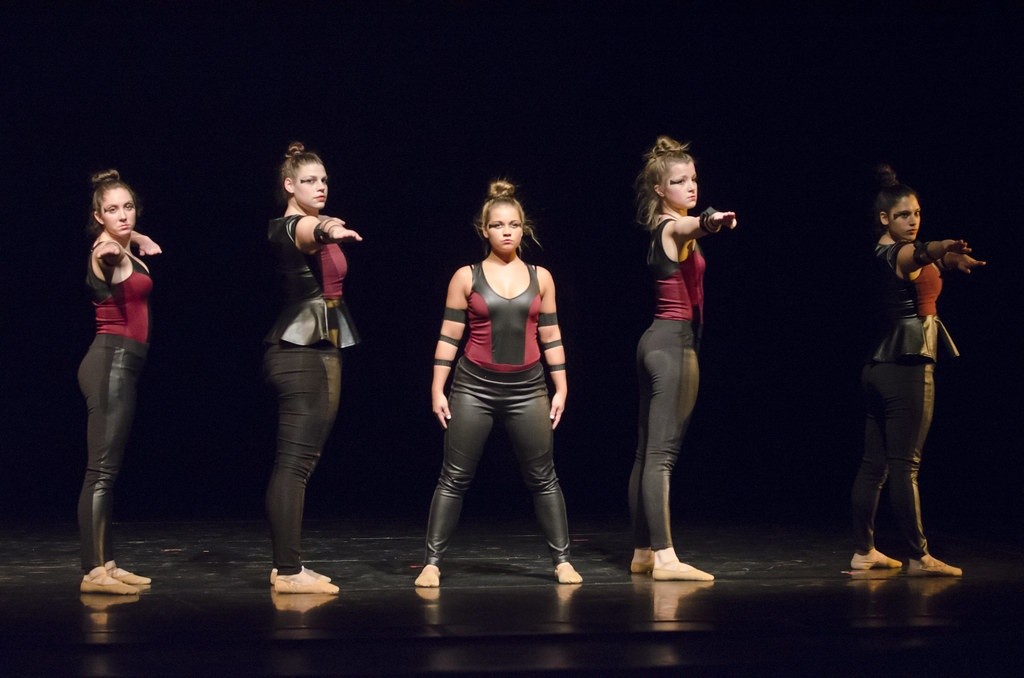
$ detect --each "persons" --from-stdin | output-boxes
[413,178,583,586]
[78,169,162,594]
[263,140,363,595]
[628,135,737,582]
[847,165,988,576]
